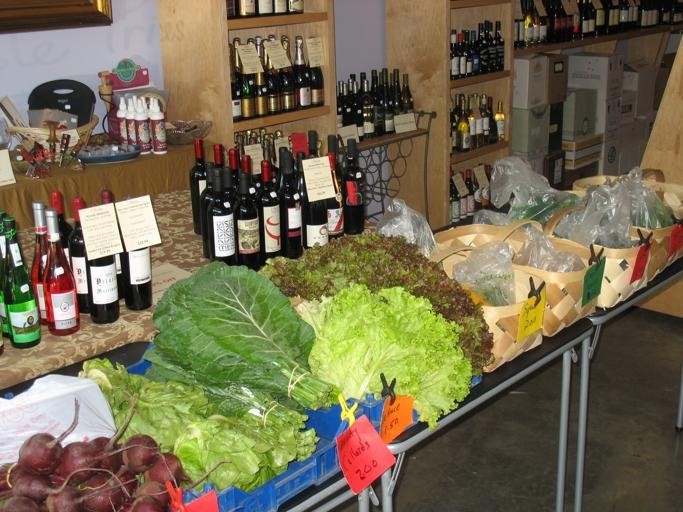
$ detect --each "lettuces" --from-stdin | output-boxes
[297,283,473,435]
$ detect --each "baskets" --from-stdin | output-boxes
[440,220,545,376]
[425,215,600,338]
[7,112,100,158]
[571,164,681,281]
[4,330,485,511]
[547,206,657,313]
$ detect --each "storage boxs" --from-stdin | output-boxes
[513,33,670,187]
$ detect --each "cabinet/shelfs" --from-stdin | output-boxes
[223,1,329,131]
[449,0,513,223]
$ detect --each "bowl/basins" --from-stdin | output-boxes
[163,120,211,145]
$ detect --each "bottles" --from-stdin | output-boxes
[189,128,364,270]
[448,162,492,223]
[513,1,683,49]
[0,188,153,354]
[115,95,167,157]
[336,66,414,142]
[228,34,325,122]
[225,0,304,20]
[446,92,506,158]
[449,20,504,80]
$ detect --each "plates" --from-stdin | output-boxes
[75,144,141,165]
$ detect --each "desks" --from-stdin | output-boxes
[0,131,214,237]
[278,257,683,512]
[0,190,212,389]
